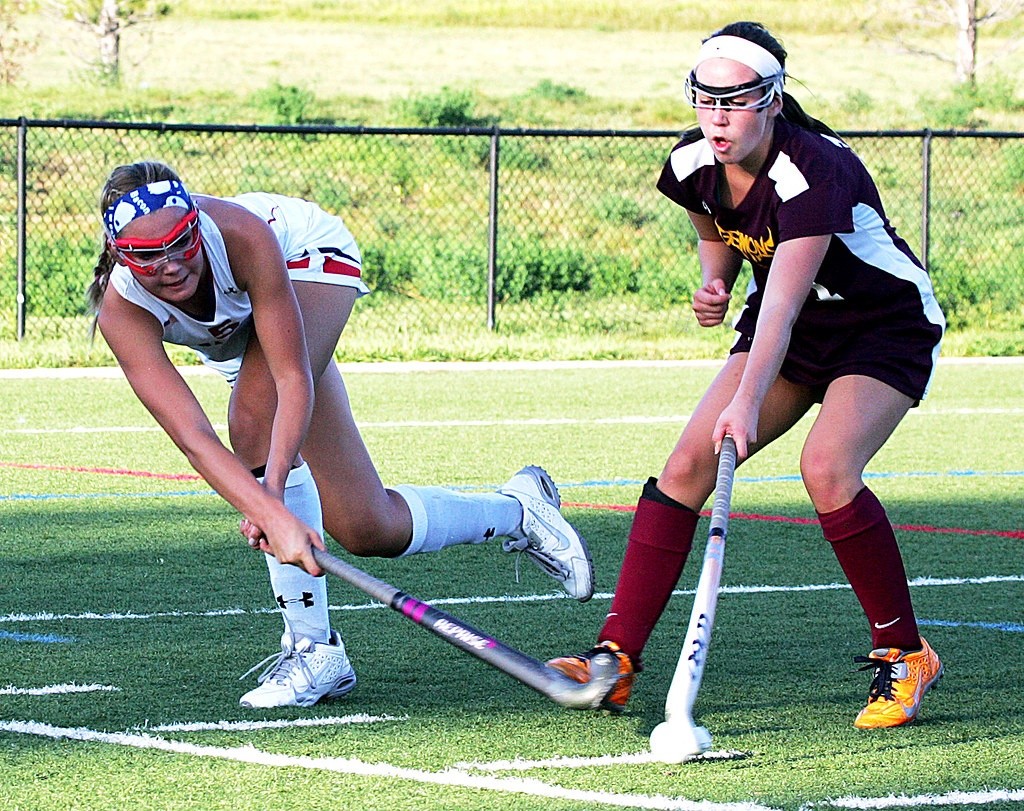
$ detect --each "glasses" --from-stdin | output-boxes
[685,67,783,109]
[114,201,202,276]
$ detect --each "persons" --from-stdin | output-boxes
[544,21,944,729]
[97,163,595,707]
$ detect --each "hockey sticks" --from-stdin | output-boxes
[247,520,619,707]
[667,435,735,750]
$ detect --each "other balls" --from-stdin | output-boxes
[651,723,689,765]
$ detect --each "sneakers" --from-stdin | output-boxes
[548,641,633,711]
[854,637,944,729]
[240,630,356,708]
[498,466,596,603]
